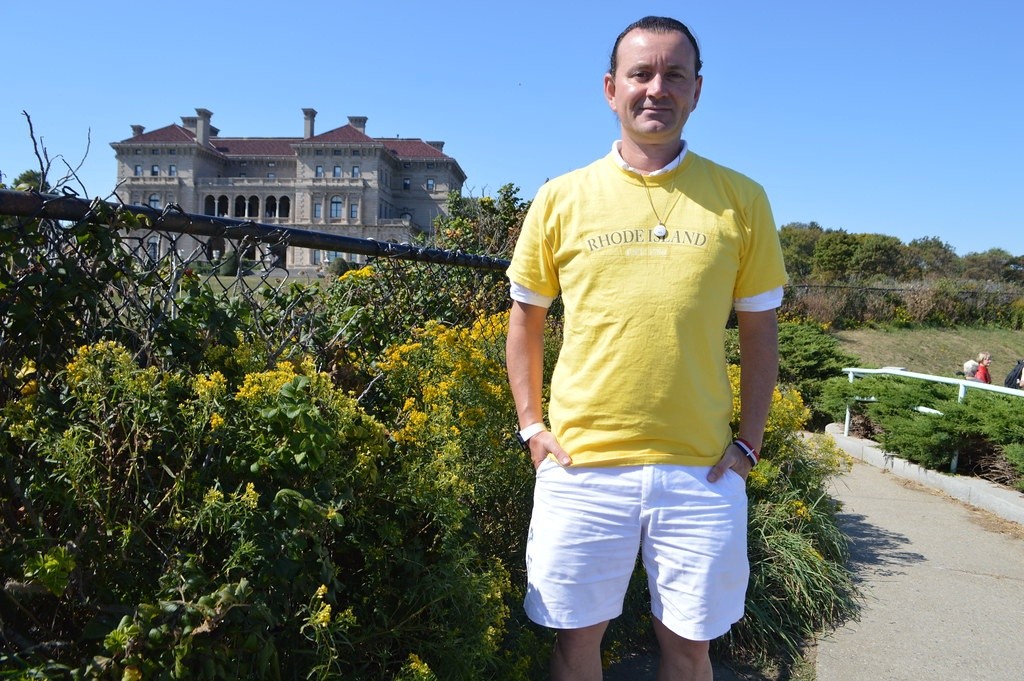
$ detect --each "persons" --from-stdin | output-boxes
[1003,359,1024,389]
[505,16,791,681]
[973,350,992,384]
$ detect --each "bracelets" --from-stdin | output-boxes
[733,437,760,467]
[519,423,547,441]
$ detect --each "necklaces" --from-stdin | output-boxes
[624,150,680,237]
